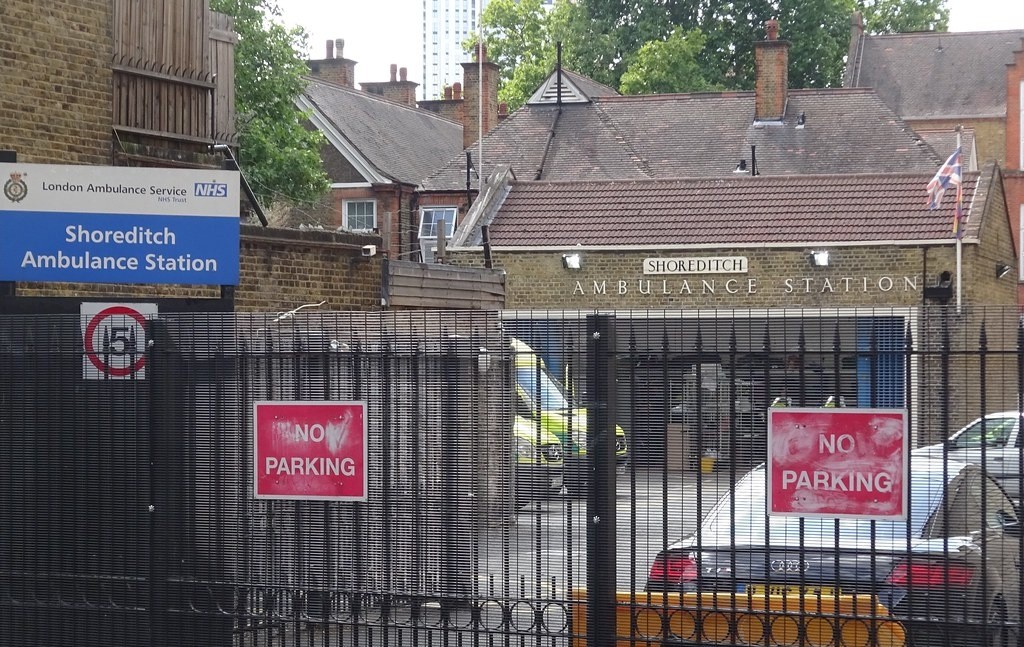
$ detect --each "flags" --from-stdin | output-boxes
[926,148,967,210]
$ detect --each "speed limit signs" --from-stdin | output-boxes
[80,302,159,380]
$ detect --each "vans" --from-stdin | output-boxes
[507,414,563,499]
[504,338,629,490]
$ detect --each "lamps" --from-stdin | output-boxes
[810,251,829,266]
[996,262,1010,279]
[733,159,751,174]
[562,253,582,270]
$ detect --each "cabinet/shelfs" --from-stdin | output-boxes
[667,423,693,471]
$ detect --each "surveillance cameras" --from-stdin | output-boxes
[362,244,377,256]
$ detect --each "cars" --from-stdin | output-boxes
[642,451,1024,647]
[917,411,1024,499]
